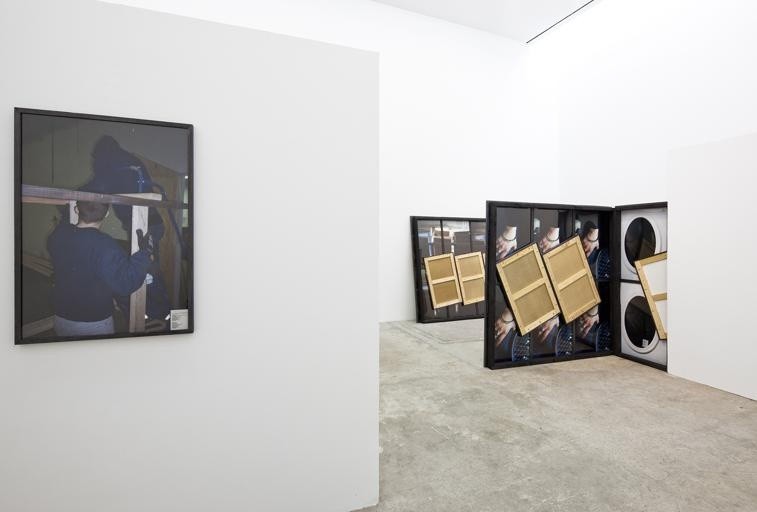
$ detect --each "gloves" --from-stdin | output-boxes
[135,227,157,256]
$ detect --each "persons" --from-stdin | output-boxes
[580,214,608,258]
[90,132,169,323]
[495,305,517,360]
[536,210,565,254]
[44,187,154,339]
[495,208,519,259]
[537,316,558,353]
[580,304,599,351]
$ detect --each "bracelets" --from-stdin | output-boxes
[545,235,559,242]
[586,311,598,317]
[499,315,514,324]
[585,235,599,242]
[501,233,517,242]
